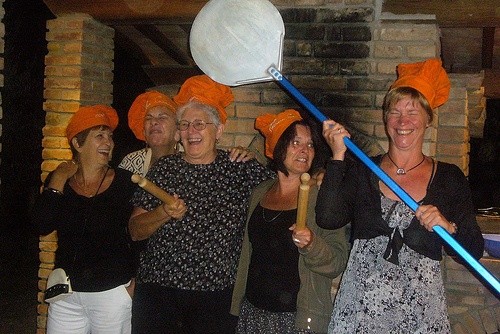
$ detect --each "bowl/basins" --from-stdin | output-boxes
[482,234,500,257]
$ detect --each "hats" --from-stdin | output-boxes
[66,106,119,141]
[128,91,178,140]
[255,109,303,159]
[389,58,451,111]
[173,75,234,124]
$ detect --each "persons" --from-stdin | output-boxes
[34,104,141,334]
[117,90,256,178]
[129,75,324,334]
[316,58,484,334]
[230,109,348,334]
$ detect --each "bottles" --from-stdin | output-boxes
[476,207,500,215]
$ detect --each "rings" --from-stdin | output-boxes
[338,128,341,132]
[71,160,75,163]
[293,239,301,243]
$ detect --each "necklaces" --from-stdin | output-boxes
[73,168,110,197]
[387,151,426,175]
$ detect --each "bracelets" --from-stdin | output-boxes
[449,221,457,235]
[44,186,62,194]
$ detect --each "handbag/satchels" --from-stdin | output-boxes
[45,268,73,303]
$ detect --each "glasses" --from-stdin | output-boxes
[176,119,216,131]
